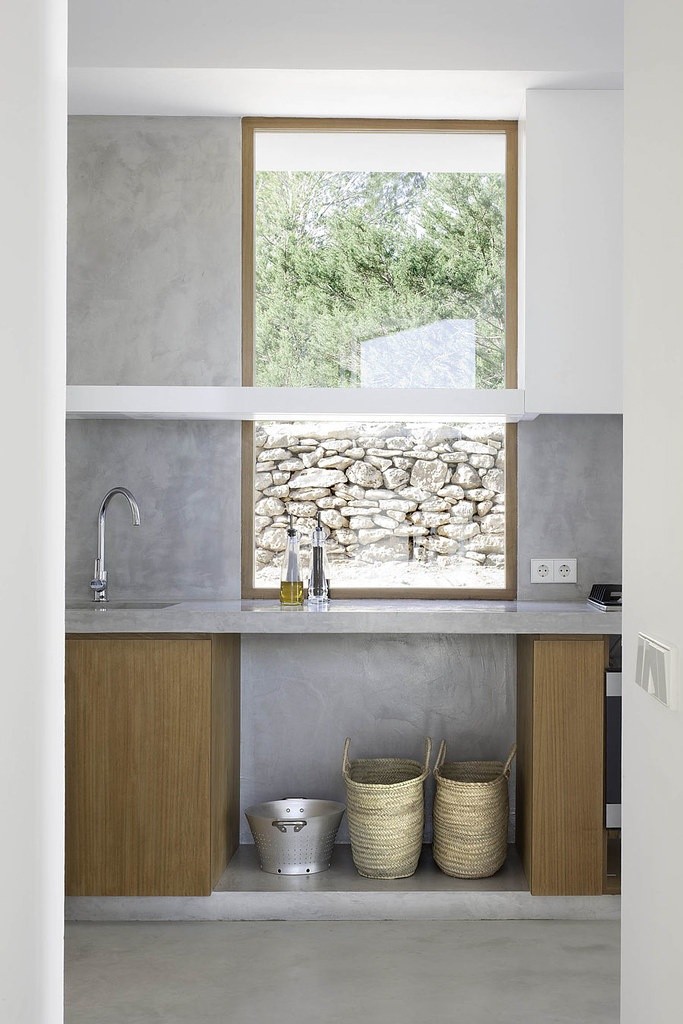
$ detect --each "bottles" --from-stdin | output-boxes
[307,527,331,601]
[279,527,305,606]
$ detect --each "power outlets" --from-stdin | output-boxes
[530,559,577,584]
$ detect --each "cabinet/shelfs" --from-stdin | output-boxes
[516,634,623,897]
[65,633,241,896]
[517,90,623,415]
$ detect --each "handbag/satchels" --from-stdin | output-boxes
[432,738,519,879]
[342,736,431,877]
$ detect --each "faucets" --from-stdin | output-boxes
[90,486,141,602]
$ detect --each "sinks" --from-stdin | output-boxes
[66,601,182,609]
